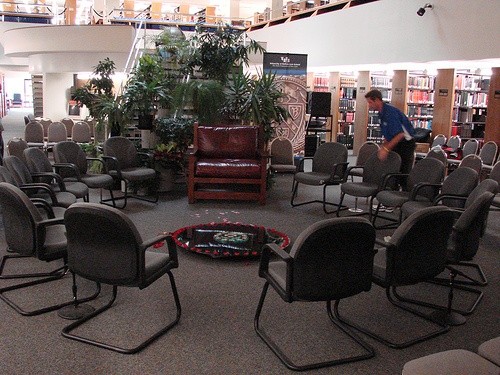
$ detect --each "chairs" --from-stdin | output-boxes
[10,94,22,105]
[255,134,500,375]
[0,113,180,355]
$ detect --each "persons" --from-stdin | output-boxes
[146,13,151,19]
[119,12,123,18]
[365,90,417,212]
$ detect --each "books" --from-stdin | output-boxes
[314,76,329,92]
[364,74,393,145]
[336,76,357,150]
[451,74,491,147]
[405,75,437,131]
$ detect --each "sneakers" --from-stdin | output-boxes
[372,204,394,212]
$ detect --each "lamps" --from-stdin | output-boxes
[417,4,432,16]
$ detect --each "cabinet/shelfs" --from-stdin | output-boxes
[162,5,189,22]
[306,67,500,152]
[119,0,135,19]
[135,2,162,19]
[190,7,216,23]
[63,0,76,25]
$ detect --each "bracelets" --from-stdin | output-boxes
[383,146,390,152]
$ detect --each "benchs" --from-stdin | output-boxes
[187,123,267,205]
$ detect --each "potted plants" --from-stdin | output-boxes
[72,8,290,197]
